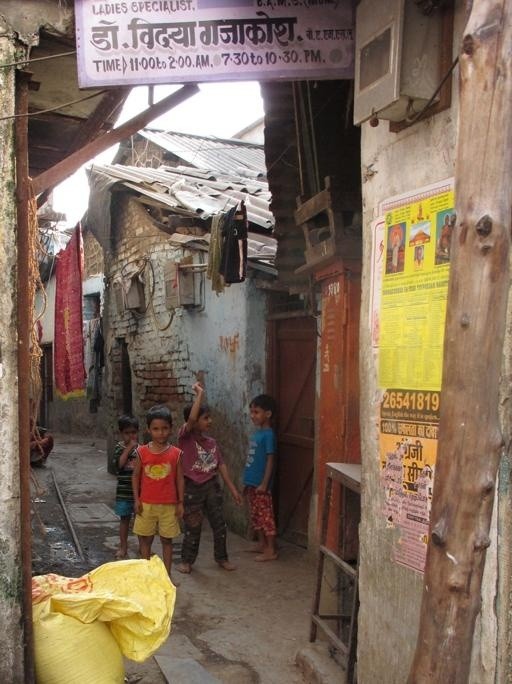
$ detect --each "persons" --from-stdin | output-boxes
[110,381,236,587]
[240,393,279,563]
